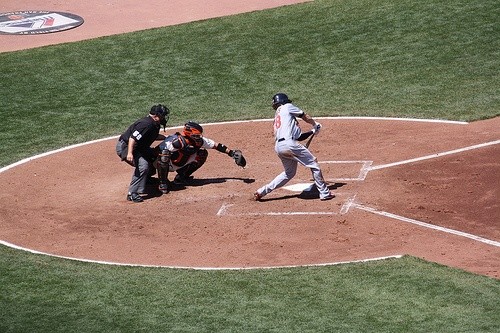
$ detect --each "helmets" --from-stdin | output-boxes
[183,122,203,144]
[272,93,292,108]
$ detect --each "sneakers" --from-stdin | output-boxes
[127,192,143,202]
[253,191,261,201]
[320,193,335,200]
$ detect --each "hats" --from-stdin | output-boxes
[150,104,169,116]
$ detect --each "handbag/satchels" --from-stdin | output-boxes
[120,143,127,161]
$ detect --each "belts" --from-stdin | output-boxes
[278,136,294,142]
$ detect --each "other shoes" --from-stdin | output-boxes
[174,174,194,185]
[158,181,168,193]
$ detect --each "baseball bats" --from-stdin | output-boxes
[305,123,320,149]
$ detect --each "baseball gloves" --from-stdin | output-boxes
[233,149,246,166]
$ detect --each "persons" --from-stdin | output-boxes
[116,104,170,203]
[254,93,335,201]
[155,121,246,194]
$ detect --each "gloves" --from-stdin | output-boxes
[311,130,320,135]
[312,122,322,130]
